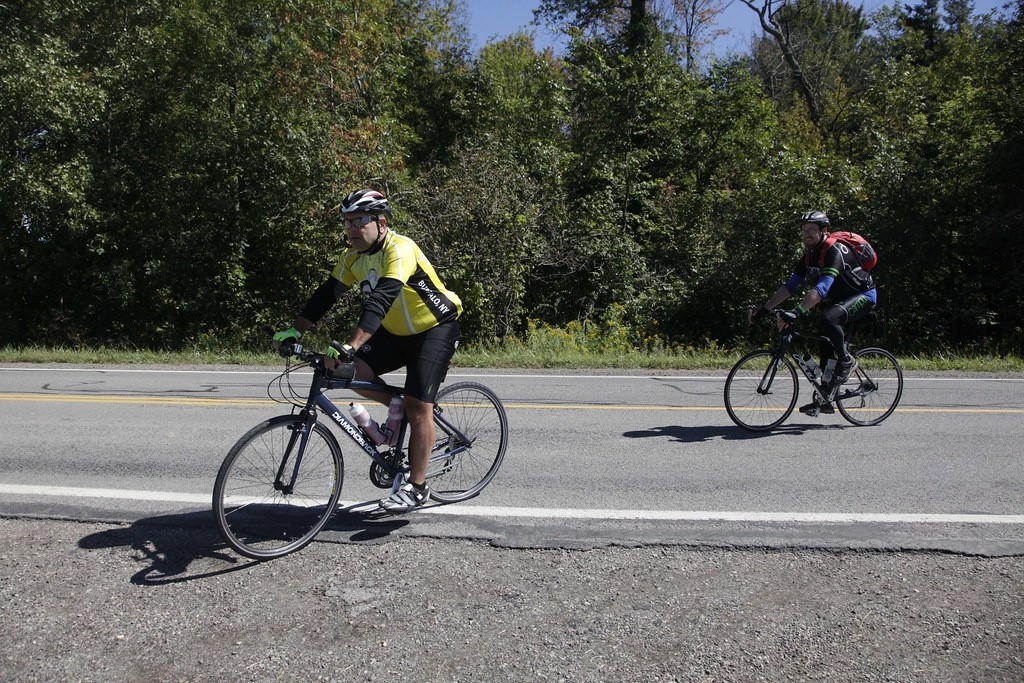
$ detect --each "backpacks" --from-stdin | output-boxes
[819,231,878,273]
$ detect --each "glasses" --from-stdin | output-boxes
[342,218,376,229]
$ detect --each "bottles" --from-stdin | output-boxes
[384,395,404,445]
[822,356,837,382]
[802,351,822,379]
[347,401,386,445]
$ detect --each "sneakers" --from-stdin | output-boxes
[380,481,431,512]
[798,403,835,414]
[833,355,858,386]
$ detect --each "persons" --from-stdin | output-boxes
[273,187,464,516]
[746,210,879,412]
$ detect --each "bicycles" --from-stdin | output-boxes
[723,305,904,434]
[212,335,510,562]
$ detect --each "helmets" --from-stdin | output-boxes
[339,188,392,219]
[799,212,829,226]
